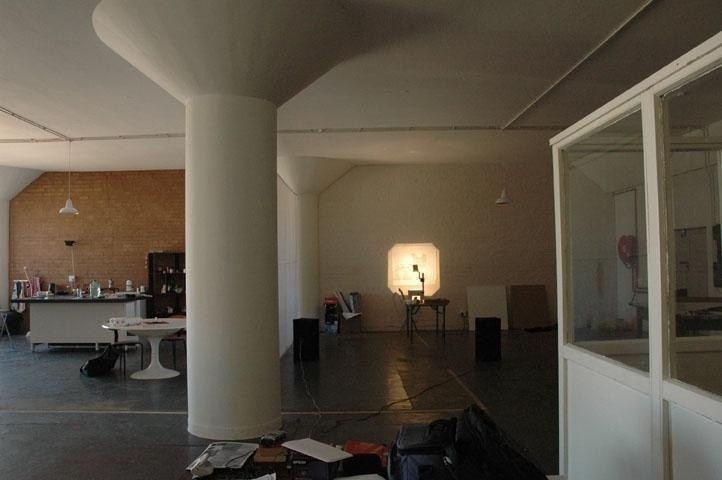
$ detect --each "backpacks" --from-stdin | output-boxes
[386,404,549,480]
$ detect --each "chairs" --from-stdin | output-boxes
[160,315,185,364]
[111,330,144,376]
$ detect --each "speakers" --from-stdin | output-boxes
[292,317,320,362]
[474,316,502,362]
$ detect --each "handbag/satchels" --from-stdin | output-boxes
[80,343,122,379]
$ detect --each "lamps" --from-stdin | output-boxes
[387,242,441,298]
[412,264,424,295]
[495,163,513,205]
[58,139,79,215]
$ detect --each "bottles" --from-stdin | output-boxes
[108,279,112,288]
[89,280,100,298]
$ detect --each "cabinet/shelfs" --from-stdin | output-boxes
[146,252,186,336]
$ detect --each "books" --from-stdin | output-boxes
[333,287,361,314]
[254,446,288,463]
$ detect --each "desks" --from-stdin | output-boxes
[10,293,153,352]
[101,318,185,380]
[405,298,449,345]
